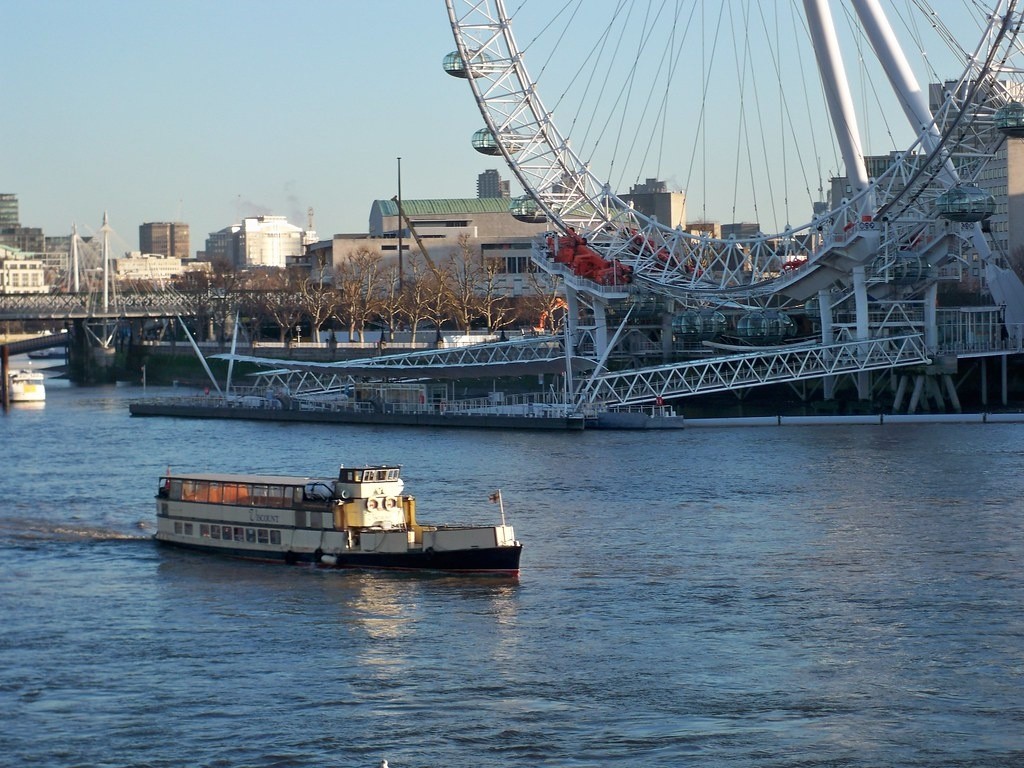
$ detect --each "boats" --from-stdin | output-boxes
[152,459,523,576]
[7,368,46,403]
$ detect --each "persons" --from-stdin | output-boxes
[543,220,707,293]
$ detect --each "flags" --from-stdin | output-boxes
[488,491,500,505]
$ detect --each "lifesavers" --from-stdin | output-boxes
[367,496,396,511]
